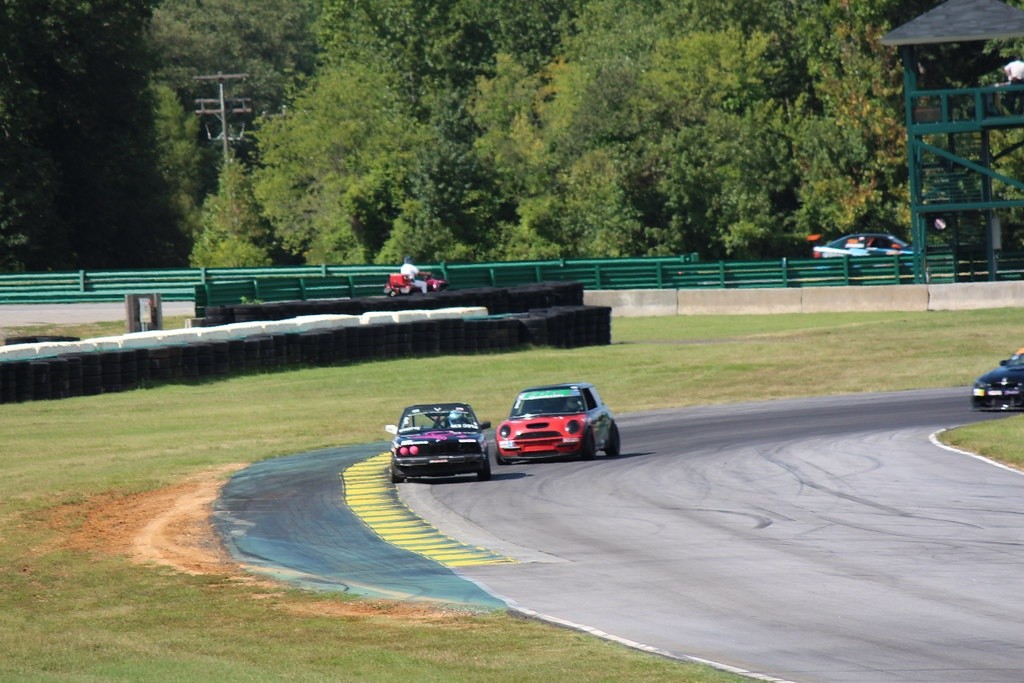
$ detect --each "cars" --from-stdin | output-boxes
[812,232,912,263]
[495,383,621,460]
[384,272,447,296]
[972,348,1024,412]
[382,401,492,478]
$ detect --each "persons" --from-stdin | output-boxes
[996,55,1024,113]
[401,256,431,295]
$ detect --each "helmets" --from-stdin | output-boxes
[404,255,416,265]
